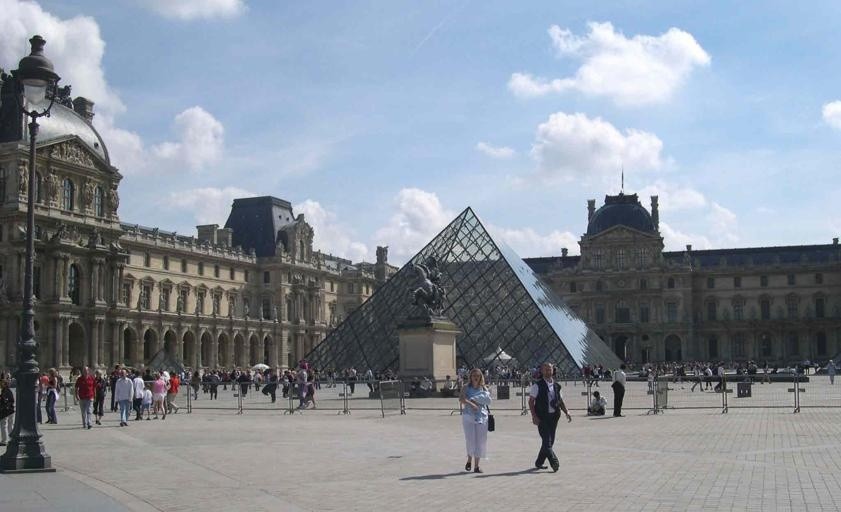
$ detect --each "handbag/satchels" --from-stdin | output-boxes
[488,415,494,432]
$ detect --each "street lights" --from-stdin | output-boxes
[0,34,64,476]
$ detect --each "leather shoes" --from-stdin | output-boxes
[465,463,470,471]
[473,468,481,473]
